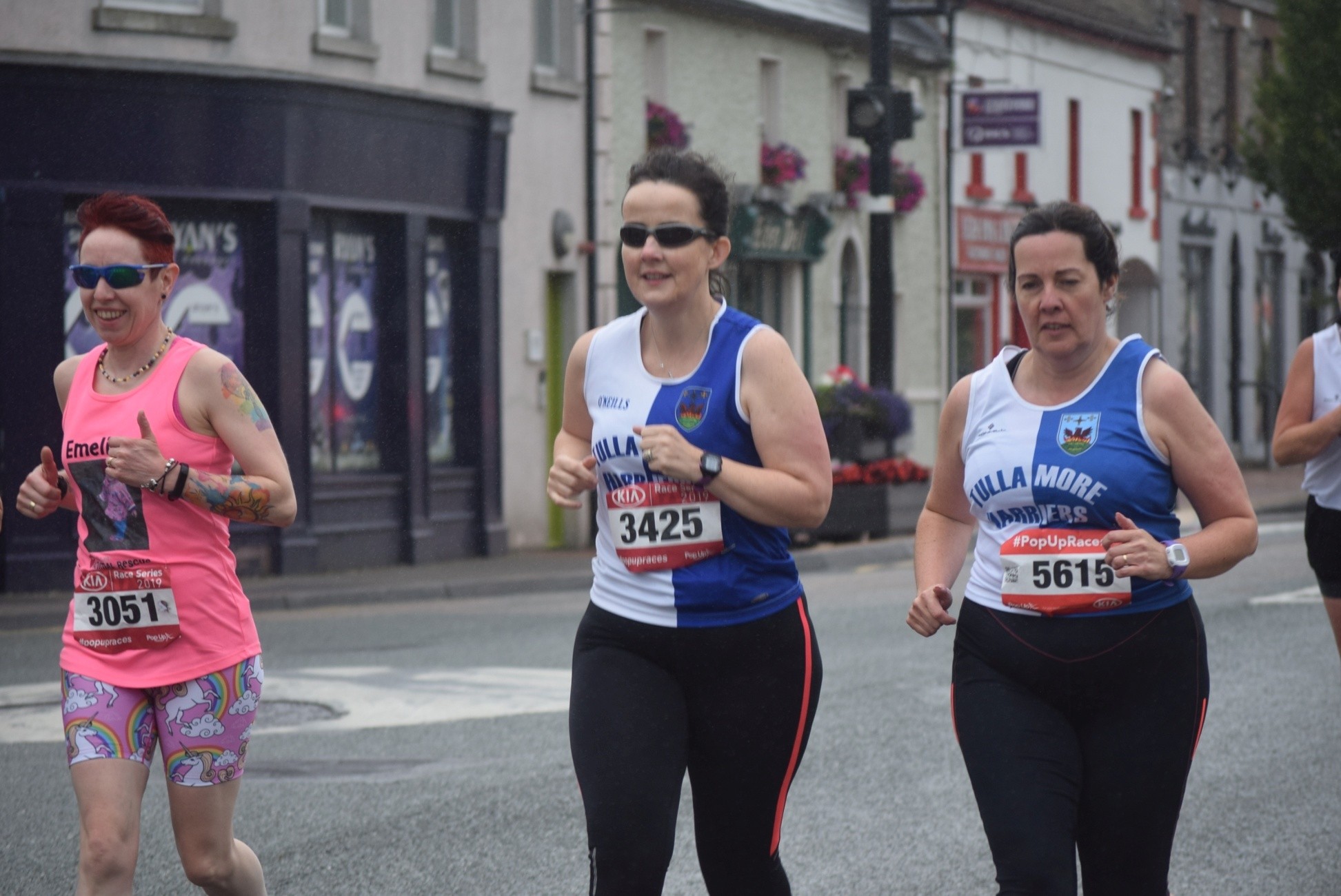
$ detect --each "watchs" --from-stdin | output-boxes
[1161,540,1190,580]
[691,450,722,491]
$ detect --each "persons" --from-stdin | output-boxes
[1272,286,1341,655]
[546,149,830,896]
[14,193,297,896]
[905,201,1258,896]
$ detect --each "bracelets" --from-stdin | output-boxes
[57,476,67,500]
[168,463,189,502]
[141,458,178,496]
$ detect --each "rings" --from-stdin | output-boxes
[30,501,35,511]
[1123,555,1128,566]
[107,457,114,468]
[648,448,652,462]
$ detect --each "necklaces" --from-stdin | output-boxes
[98,326,171,383]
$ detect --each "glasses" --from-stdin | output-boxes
[69,265,158,290]
[620,224,712,249]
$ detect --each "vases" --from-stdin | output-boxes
[811,478,934,545]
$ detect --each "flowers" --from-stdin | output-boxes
[760,138,805,187]
[831,148,926,212]
[645,98,690,155]
[804,364,935,485]
